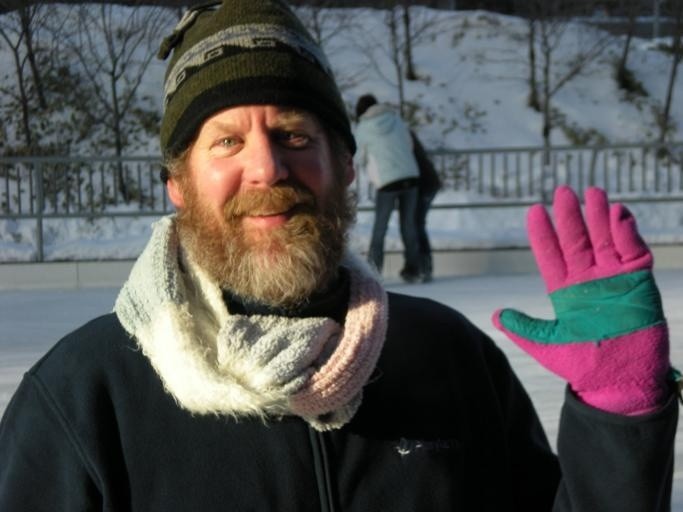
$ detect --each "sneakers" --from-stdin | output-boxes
[397,270,433,282]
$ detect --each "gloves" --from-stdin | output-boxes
[490,182,674,415]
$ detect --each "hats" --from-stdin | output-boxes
[160,1,357,182]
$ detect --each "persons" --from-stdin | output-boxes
[352,91,424,286]
[0,3,681,512]
[399,125,442,283]
